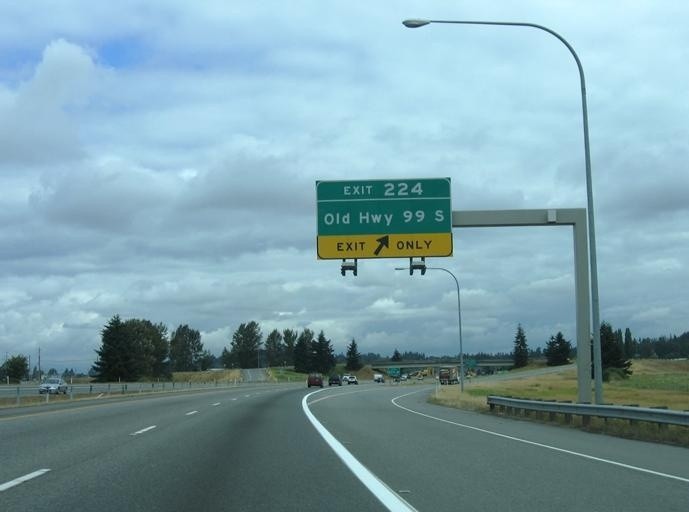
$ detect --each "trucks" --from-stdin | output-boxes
[439,366,460,385]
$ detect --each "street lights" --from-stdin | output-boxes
[397,266,465,389]
[401,18,603,407]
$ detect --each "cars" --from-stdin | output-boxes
[36,375,70,396]
[399,374,410,381]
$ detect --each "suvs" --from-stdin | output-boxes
[304,371,342,393]
[348,376,358,387]
[343,374,350,382]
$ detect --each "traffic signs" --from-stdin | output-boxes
[316,178,451,256]
